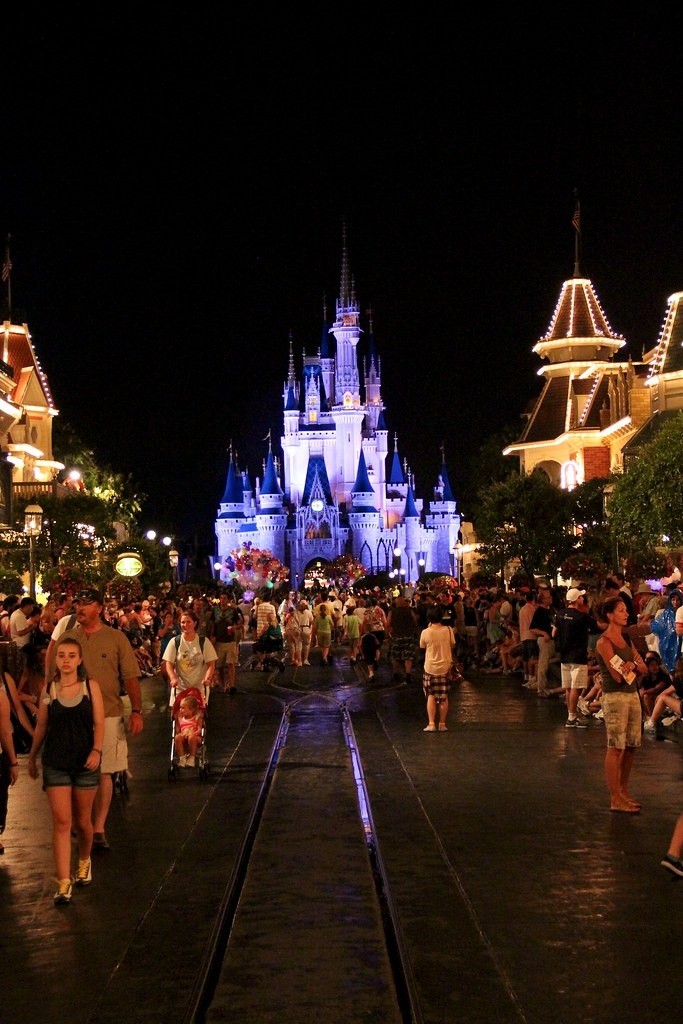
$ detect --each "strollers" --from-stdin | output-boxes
[166,683,211,785]
[249,637,286,672]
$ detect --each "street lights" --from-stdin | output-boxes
[168,547,180,585]
[146,528,172,585]
[24,494,43,603]
[388,547,426,587]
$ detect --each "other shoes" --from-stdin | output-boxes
[211,657,412,695]
[139,665,162,678]
[476,660,679,734]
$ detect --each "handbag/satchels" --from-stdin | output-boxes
[448,663,461,681]
[485,623,506,644]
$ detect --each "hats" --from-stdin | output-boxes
[633,584,657,595]
[148,595,156,600]
[71,587,103,604]
[565,588,586,603]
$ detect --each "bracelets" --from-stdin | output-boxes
[92,748,102,755]
[633,661,638,667]
[11,761,18,766]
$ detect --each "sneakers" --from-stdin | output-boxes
[565,715,588,729]
[438,725,448,732]
[53,877,76,904]
[93,831,109,851]
[75,858,92,887]
[71,824,82,839]
[423,725,437,732]
[660,855,683,878]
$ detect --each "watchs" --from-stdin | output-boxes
[133,708,143,714]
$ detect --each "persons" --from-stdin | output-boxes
[661,812,683,879]
[237,586,362,671]
[0,593,244,904]
[389,582,490,731]
[486,574,638,729]
[338,586,392,679]
[594,582,683,812]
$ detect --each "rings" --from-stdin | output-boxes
[629,667,632,669]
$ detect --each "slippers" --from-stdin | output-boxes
[608,798,642,815]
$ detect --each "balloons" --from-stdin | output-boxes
[221,540,290,590]
[646,578,656,586]
[242,590,255,601]
[651,580,661,591]
[324,552,367,586]
